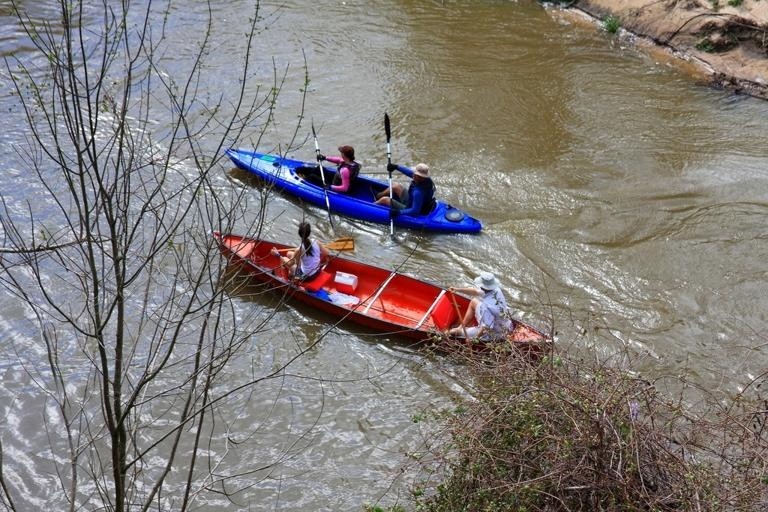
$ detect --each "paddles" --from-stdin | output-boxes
[310,117,332,228]
[384,112,394,238]
[272,236,355,252]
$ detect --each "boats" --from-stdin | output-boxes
[212,230,555,366]
[226,149,481,235]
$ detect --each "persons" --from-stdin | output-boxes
[369,163,436,218]
[446,271,514,341]
[270,221,322,283]
[317,146,362,194]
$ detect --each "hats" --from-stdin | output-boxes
[413,163,430,178]
[474,272,499,291]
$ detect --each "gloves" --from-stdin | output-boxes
[318,155,325,161]
[389,209,400,216]
[387,163,397,171]
[321,184,331,189]
